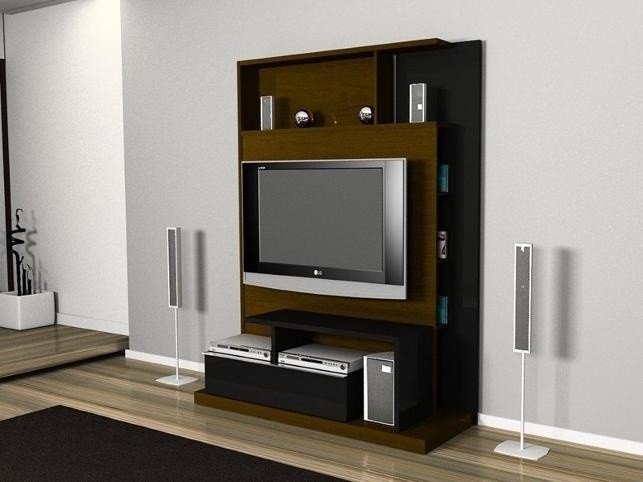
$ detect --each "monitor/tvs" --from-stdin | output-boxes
[240,157,407,301]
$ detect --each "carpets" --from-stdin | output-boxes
[1,404,350,481]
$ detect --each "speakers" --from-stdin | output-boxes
[166,227,182,308]
[260,95,275,130]
[409,83,427,123]
[363,352,395,427]
[513,243,532,353]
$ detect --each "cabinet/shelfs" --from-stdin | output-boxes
[192,38,483,456]
